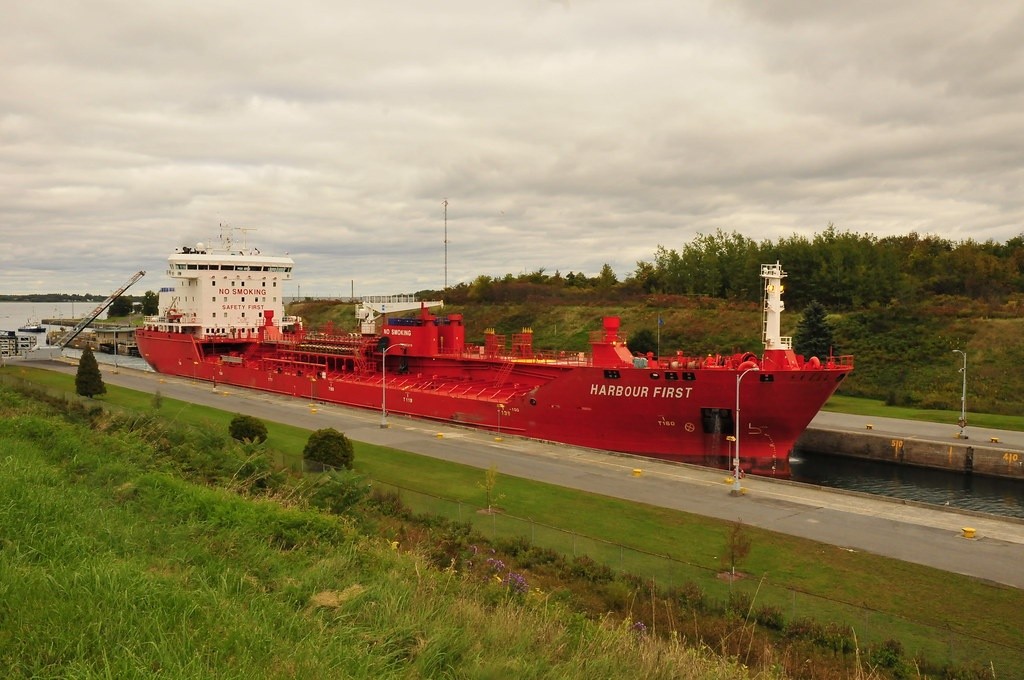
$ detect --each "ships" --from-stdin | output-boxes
[132,222,855,482]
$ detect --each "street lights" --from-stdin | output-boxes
[950,348,965,435]
[381,342,412,427]
[734,366,764,480]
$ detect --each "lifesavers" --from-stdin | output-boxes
[191,317,196,323]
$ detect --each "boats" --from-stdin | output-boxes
[18,317,47,332]
[46,327,141,357]
[0,270,146,364]
